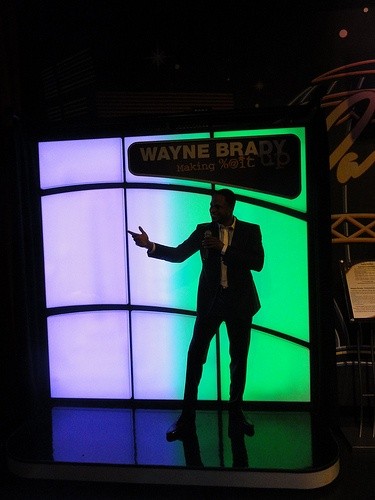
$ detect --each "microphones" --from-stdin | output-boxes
[204,230,211,254]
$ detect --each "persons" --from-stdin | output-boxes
[128,188,265,439]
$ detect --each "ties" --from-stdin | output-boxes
[221,229,229,287]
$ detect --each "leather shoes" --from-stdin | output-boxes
[166,423,196,438]
[232,414,253,429]
